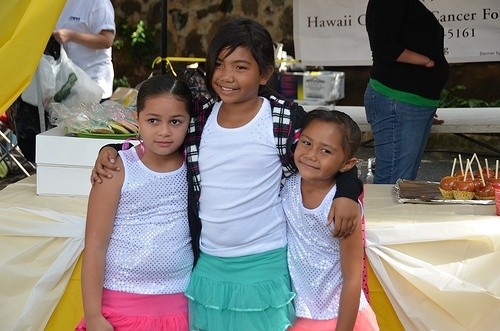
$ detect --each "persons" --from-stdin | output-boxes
[279,106,380,331]
[52,0,116,104]
[74,74,195,331]
[89,16,364,331]
[363,0,451,186]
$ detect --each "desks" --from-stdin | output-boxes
[301,107,499,157]
[0,174,500,331]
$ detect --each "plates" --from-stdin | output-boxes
[76,131,140,139]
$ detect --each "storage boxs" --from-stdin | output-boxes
[36,127,143,196]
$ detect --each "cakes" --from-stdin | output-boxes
[438,167,500,200]
